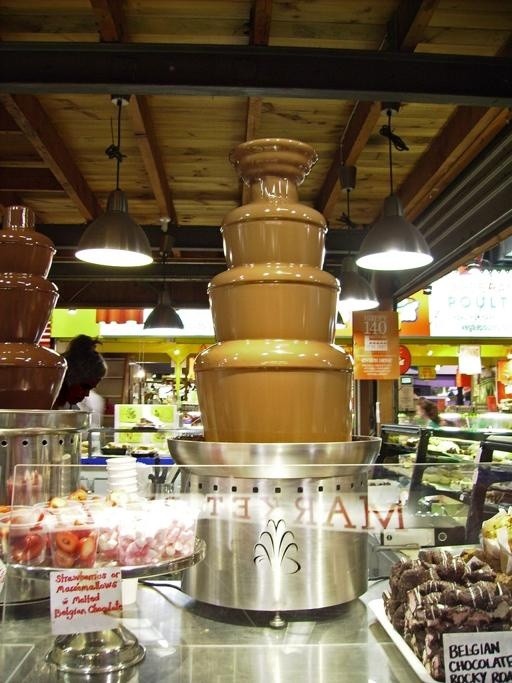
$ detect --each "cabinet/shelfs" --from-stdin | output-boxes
[375,423,512,544]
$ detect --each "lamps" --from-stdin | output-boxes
[336,312,346,329]
[329,167,379,308]
[144,210,185,332]
[351,102,434,270]
[71,84,155,267]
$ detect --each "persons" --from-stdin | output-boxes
[414,398,442,429]
[51,334,108,410]
